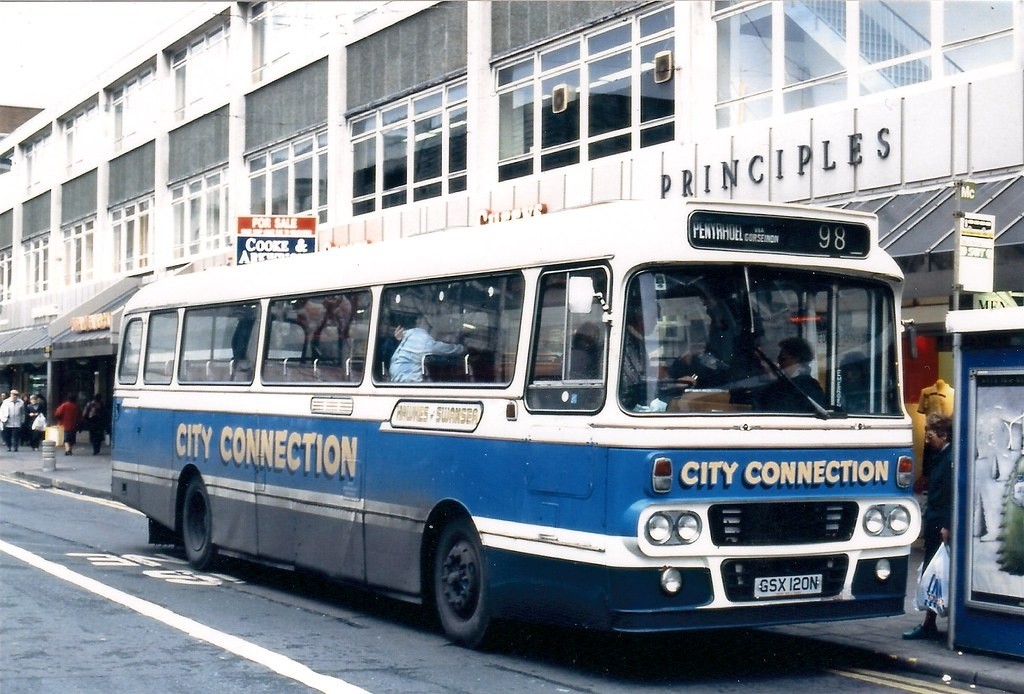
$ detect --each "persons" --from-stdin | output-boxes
[80,393,110,455]
[557,322,601,408]
[915,378,955,477]
[902,417,955,639]
[618,297,699,414]
[379,300,474,384]
[0,389,26,452]
[670,274,757,390]
[54,392,84,456]
[0,390,47,446]
[26,394,44,451]
[751,337,826,415]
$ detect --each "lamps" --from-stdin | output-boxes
[44,344,53,359]
[653,50,680,84]
[552,82,578,113]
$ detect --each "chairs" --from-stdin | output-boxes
[150,349,565,382]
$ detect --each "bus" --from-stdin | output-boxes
[108,197,923,649]
